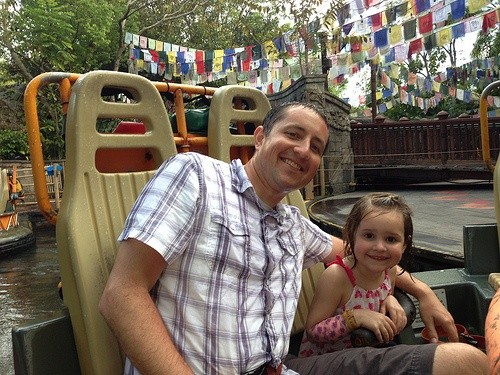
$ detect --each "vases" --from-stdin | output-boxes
[421,323,487,353]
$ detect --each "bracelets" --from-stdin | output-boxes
[343,310,358,330]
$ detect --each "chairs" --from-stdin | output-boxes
[55,70,417,375]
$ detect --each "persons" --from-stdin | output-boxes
[298,192,413,359]
[3,170,24,213]
[97,102,488,375]
[483,153,500,374]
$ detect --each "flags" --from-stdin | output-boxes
[124,0,500,119]
